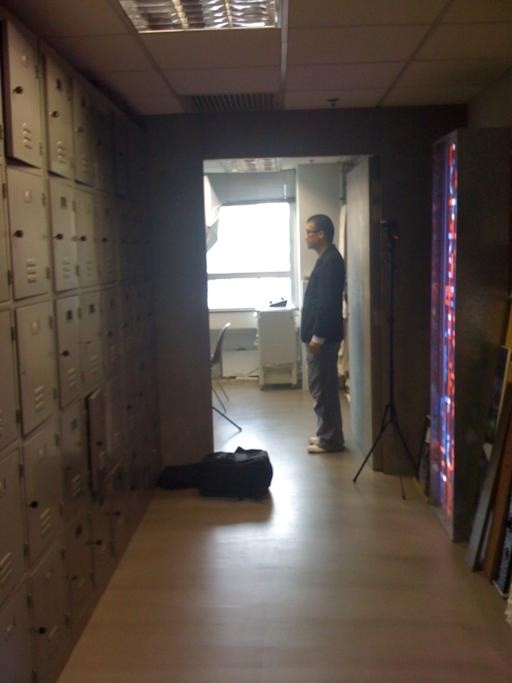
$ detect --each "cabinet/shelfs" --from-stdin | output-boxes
[255,299,299,391]
[2,1,161,683]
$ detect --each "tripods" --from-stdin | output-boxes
[353,239,419,500]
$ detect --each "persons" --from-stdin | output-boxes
[299,213,350,455]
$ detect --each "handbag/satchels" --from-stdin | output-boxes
[198,445,274,498]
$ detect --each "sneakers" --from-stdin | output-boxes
[305,435,328,453]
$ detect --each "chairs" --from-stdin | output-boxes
[210,322,231,414]
